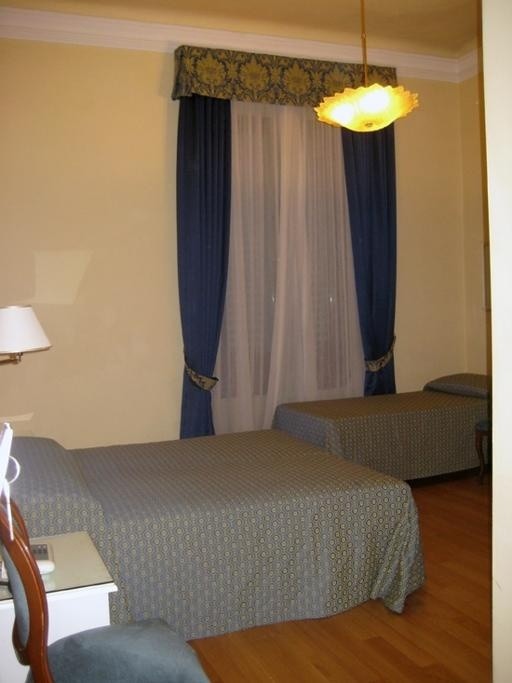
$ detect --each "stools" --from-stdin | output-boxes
[476,418,492,484]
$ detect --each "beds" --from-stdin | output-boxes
[271,373,492,484]
[0,427,427,642]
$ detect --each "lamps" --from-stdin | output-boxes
[313,1,420,133]
[0,305,51,367]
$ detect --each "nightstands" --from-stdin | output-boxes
[0,529,120,683]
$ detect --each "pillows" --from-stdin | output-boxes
[423,374,492,399]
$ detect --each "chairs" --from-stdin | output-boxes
[0,491,212,682]
